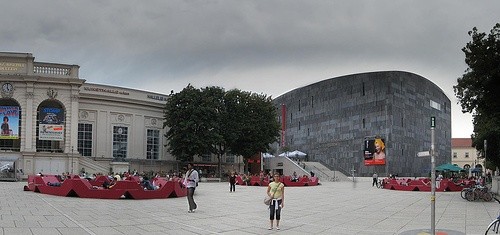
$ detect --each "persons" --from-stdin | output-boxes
[372,172,378,186]
[373,139,385,160]
[229,171,235,192]
[182,163,199,212]
[39,170,203,190]
[267,172,285,231]
[0,116,11,136]
[235,172,315,186]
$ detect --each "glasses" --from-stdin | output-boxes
[274,176,281,178]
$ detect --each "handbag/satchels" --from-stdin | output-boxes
[264,194,273,205]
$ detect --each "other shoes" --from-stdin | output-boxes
[276,226,281,230]
[268,226,273,230]
[188,207,196,213]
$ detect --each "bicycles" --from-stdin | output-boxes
[376,178,384,189]
[461,181,493,202]
[485,197,500,235]
